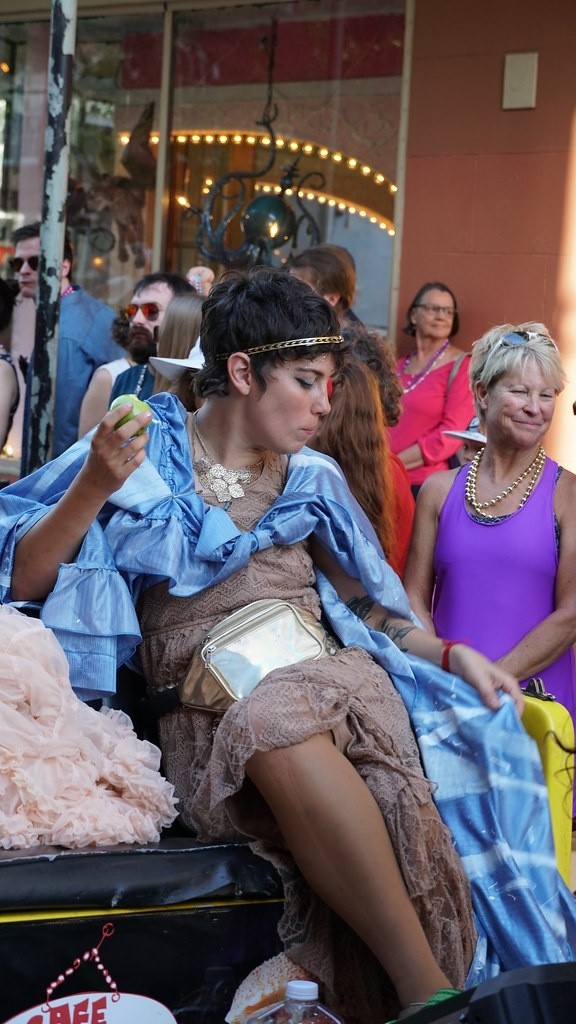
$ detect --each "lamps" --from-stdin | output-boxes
[192,1,326,275]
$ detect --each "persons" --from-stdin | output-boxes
[385,280,475,503]
[109,289,209,408]
[307,357,396,571]
[338,321,416,578]
[0,262,524,1024]
[403,322,576,726]
[0,272,20,456]
[11,220,130,481]
[303,244,368,323]
[188,249,354,324]
[78,271,196,443]
[441,415,486,461]
[151,336,206,414]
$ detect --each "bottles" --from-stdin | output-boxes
[240,980,342,1024]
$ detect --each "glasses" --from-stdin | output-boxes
[416,304,455,317]
[486,332,557,355]
[128,303,165,321]
[9,255,40,274]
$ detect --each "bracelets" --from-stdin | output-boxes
[440,638,467,672]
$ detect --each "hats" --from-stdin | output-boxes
[150,335,206,387]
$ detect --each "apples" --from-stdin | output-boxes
[108,394,152,435]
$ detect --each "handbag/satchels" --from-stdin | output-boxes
[179,597,335,718]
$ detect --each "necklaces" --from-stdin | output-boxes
[61,285,74,296]
[397,339,450,394]
[465,444,546,519]
[190,406,266,505]
